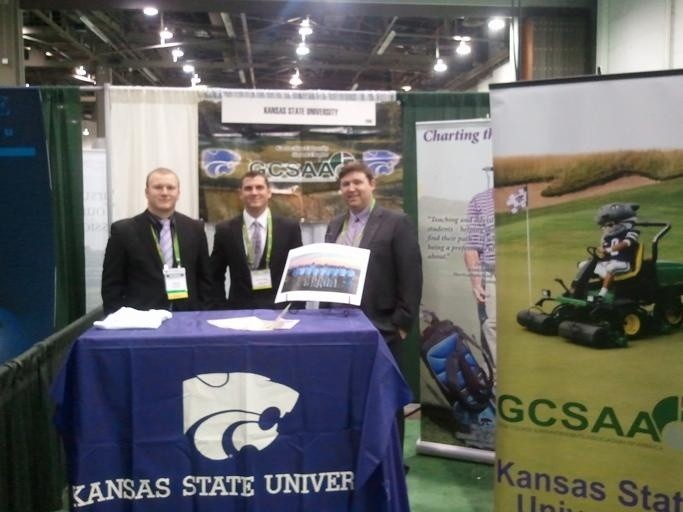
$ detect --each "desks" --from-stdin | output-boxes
[66,306,395,512]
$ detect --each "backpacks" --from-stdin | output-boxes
[418,310,495,409]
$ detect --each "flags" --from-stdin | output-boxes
[505,186,529,215]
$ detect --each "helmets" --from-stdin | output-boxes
[594,201,637,238]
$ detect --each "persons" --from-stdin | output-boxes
[577,202,640,303]
[463,189,497,372]
[209,170,309,311]
[318,160,423,474]
[100,167,209,317]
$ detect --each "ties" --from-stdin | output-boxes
[340,219,363,248]
[156,218,175,270]
[248,221,264,271]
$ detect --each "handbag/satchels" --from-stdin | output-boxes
[449,382,503,451]
